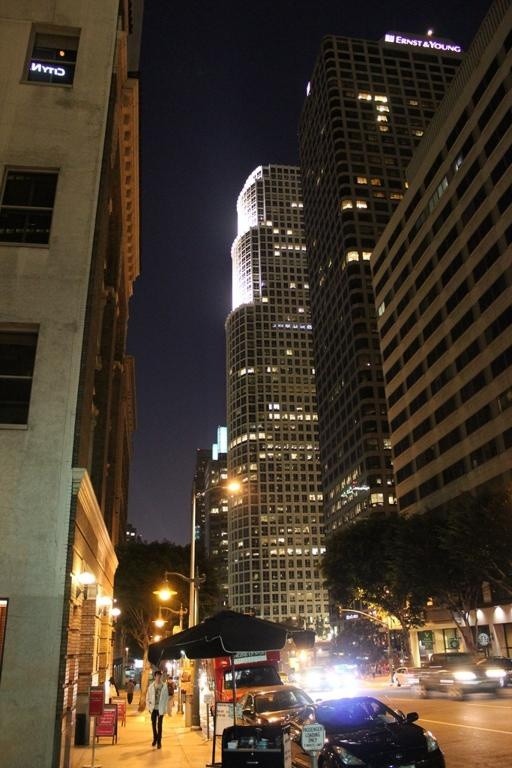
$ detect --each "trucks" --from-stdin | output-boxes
[209,651,283,702]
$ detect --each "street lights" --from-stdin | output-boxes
[189,482,241,630]
[150,565,207,727]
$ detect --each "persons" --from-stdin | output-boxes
[357,660,389,679]
[124,676,177,717]
[145,670,170,749]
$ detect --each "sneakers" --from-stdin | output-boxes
[152,740,161,749]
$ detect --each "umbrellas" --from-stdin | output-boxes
[148,608,316,726]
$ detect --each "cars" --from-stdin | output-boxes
[237,687,319,726]
[280,697,446,768]
[393,653,512,701]
